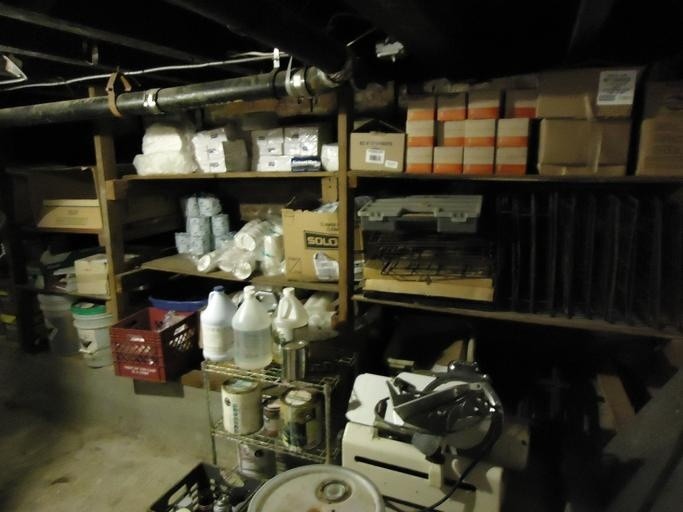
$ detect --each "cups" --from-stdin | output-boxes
[194,219,283,279]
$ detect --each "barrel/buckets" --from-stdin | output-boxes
[247,464,386,512]
[35,292,114,369]
[198,285,238,363]
[272,287,310,365]
